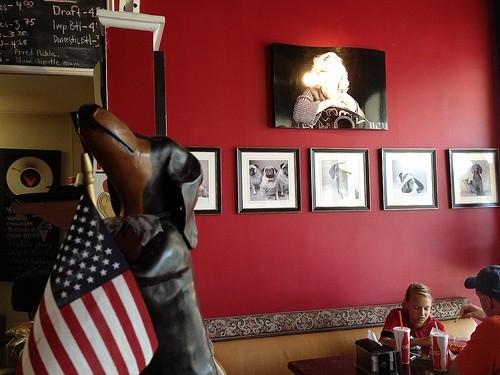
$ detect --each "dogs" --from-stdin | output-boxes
[462,163,485,195]
[398,171,424,194]
[249,162,289,200]
[76,103,226,375]
[329,162,359,199]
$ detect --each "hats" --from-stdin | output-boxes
[464,265,500,293]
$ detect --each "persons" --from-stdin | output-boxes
[294,52,365,128]
[380,283,445,346]
[447,266,500,375]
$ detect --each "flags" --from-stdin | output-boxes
[18,184,159,375]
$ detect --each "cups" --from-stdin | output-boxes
[393,327,411,364]
[74,173,83,185]
[430,332,449,372]
[68,177,75,185]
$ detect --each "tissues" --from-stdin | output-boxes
[354,330,396,371]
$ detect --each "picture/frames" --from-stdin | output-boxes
[448,147,500,209]
[310,147,371,213]
[381,147,439,211]
[237,147,302,214]
[185,146,222,216]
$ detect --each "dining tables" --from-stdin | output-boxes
[288,347,460,375]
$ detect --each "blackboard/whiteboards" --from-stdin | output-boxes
[0,0,114,77]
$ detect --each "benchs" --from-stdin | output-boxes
[204,297,478,375]
